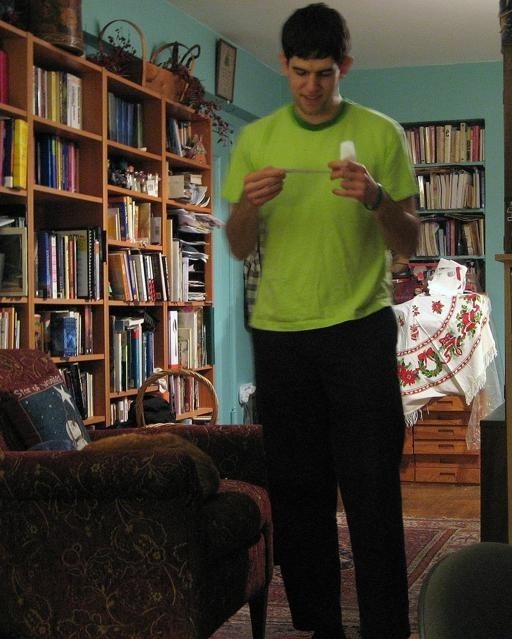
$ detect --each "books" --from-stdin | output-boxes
[405,123,485,257]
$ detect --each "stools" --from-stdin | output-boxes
[415,539,512,639]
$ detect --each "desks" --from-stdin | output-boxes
[479,396,509,542]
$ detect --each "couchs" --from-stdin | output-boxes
[0,346,276,637]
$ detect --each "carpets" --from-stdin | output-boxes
[209,505,481,638]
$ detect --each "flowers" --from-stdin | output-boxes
[87,25,137,77]
[160,58,234,147]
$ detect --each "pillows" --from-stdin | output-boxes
[0,371,95,450]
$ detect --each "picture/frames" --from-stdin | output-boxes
[215,38,238,101]
[0,227,29,299]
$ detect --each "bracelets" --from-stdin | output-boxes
[365,182,383,211]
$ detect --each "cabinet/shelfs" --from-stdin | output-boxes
[104,66,214,435]
[0,20,106,429]
[407,158,486,263]
[398,388,485,488]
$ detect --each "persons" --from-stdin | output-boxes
[219,3,422,639]
